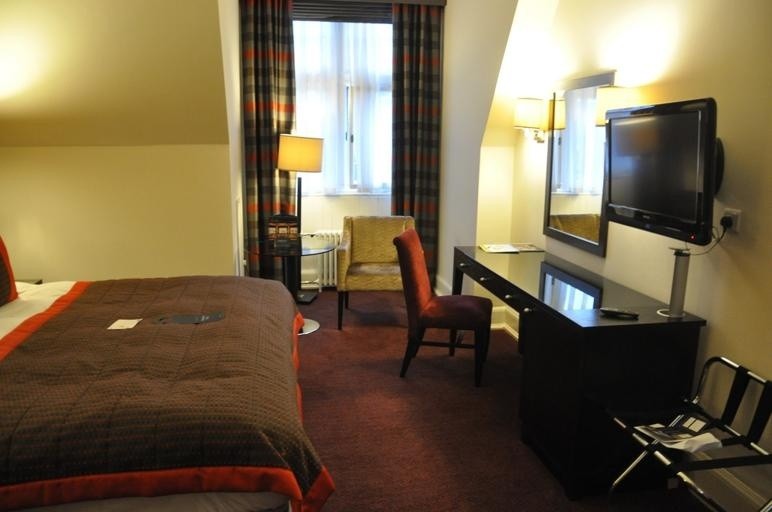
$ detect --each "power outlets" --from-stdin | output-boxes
[724,207,742,232]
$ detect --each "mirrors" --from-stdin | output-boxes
[538,261,602,310]
[543,72,616,258]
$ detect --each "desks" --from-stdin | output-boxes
[245,232,337,336]
[449,243,706,500]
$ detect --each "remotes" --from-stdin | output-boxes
[599,306,641,320]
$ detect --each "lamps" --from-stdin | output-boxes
[554,99,566,130]
[594,85,634,127]
[277,134,323,304]
[511,95,547,143]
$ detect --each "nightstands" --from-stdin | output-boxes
[15,278,44,284]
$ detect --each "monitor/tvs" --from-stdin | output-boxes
[602,96,726,247]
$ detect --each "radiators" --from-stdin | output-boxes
[300,233,341,292]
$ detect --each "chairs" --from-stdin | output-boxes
[608,355,772,511]
[336,214,415,329]
[393,230,492,386]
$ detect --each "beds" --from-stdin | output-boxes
[1,276,337,511]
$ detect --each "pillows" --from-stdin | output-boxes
[1,236,18,305]
